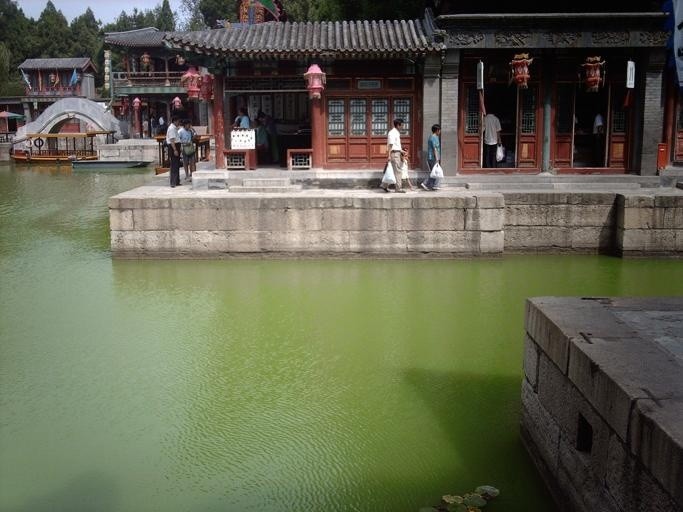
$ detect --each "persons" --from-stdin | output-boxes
[481,109,504,165]
[382,119,404,191]
[140,108,277,185]
[419,123,445,192]
[592,109,606,164]
[399,148,412,192]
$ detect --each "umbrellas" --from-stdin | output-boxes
[0,110,27,131]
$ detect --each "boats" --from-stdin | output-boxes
[7,128,119,166]
[71,160,152,170]
[153,133,213,175]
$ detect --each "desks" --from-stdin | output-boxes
[0,132,14,142]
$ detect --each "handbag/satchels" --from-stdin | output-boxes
[192,134,201,144]
[183,144,195,156]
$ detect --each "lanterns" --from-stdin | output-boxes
[175,53,187,67]
[129,96,143,111]
[172,96,180,107]
[301,63,327,104]
[181,65,215,101]
[578,55,608,90]
[510,51,533,86]
[141,50,152,65]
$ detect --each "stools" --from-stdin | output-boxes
[0,136,4,142]
